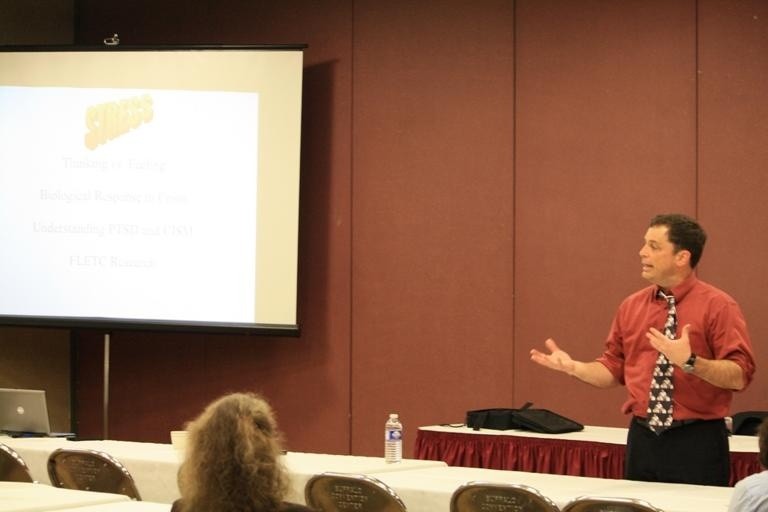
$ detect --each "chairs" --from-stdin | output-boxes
[1,443,39,483]
[47,447,140,501]
[449,481,560,511]
[304,471,407,511]
[563,496,658,511]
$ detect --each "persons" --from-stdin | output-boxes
[170,394,309,512]
[529,212,752,487]
[728,417,768,512]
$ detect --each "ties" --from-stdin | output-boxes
[646,289,679,437]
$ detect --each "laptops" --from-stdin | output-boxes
[0,388,78,439]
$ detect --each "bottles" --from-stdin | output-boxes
[383,411,405,464]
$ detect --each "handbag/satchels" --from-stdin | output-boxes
[466,402,583,434]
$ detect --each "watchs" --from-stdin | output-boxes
[681,353,698,373]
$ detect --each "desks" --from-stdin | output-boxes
[414,424,763,487]
[369,467,736,511]
[73,498,172,510]
[0,436,446,506]
[0,480,131,511]
